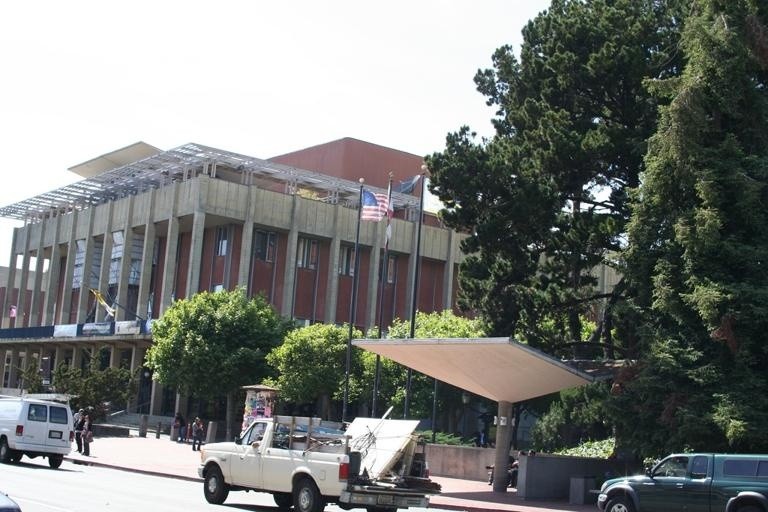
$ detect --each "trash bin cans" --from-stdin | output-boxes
[411,442,426,477]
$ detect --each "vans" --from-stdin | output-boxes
[1,394,76,468]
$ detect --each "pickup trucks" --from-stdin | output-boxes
[197,418,434,511]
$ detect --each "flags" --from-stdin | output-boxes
[91,289,115,318]
[361,187,391,224]
[8,303,17,318]
[385,189,395,246]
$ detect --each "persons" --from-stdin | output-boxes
[171,416,179,429]
[74,413,84,453]
[74,408,85,437]
[485,449,536,488]
[191,417,203,450]
[197,420,205,450]
[176,412,186,443]
[249,440,263,447]
[79,414,92,456]
[338,422,346,433]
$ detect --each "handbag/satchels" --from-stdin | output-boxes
[85,431,93,442]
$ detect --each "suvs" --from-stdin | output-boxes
[595,452,768,512]
